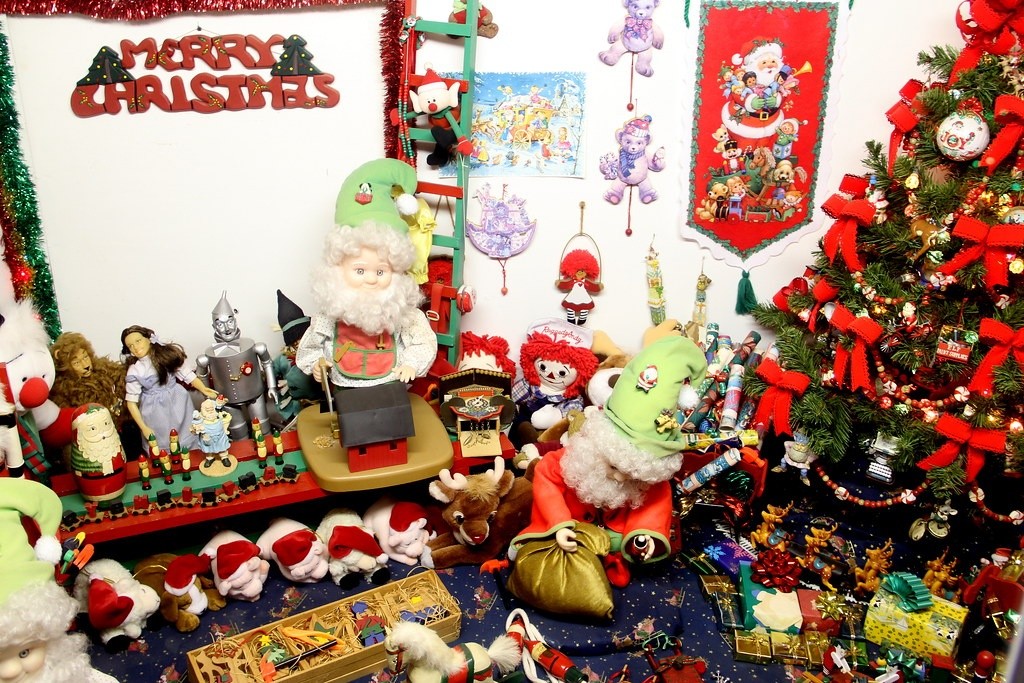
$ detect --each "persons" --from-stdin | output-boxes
[71,403,127,503]
[0,479,120,683]
[455,317,599,429]
[195,290,278,441]
[555,248,604,326]
[508,618,589,683]
[273,289,327,401]
[740,185,1024,568]
[295,158,438,397]
[351,601,387,646]
[509,334,708,588]
[121,325,219,455]
[410,62,473,165]
[138,399,282,491]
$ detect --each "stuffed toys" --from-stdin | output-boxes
[316,458,540,590]
[0,297,76,478]
[537,352,633,441]
[448,0,499,39]
[70,553,227,650]
[383,620,521,683]
[198,518,328,602]
[49,332,127,427]
